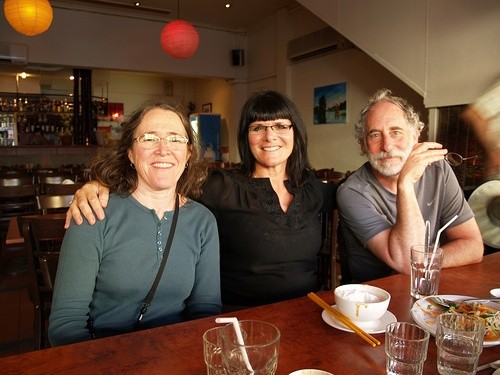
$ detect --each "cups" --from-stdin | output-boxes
[384,322,430,375]
[410,246,444,300]
[434,312,485,375]
[220,319,280,375]
[204,326,248,375]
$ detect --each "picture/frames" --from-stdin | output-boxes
[313,82,347,125]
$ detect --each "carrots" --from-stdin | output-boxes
[426,305,500,341]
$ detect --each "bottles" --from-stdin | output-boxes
[92,98,108,117]
[0,96,75,148]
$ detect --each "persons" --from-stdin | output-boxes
[48,103,224,347]
[336,88,484,283]
[64,91,341,320]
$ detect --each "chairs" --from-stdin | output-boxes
[0,160,357,351]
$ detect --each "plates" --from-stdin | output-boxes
[288,368,333,375]
[490,288,500,298]
[321,304,397,334]
[409,294,500,348]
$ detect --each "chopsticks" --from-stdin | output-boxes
[308,293,380,347]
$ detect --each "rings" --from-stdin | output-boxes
[69,201,75,205]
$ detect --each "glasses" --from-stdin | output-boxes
[131,133,189,150]
[245,124,293,134]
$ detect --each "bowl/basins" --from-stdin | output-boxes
[334,283,391,323]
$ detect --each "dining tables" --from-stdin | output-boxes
[0,251,500,375]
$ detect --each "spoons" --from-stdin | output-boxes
[418,220,433,295]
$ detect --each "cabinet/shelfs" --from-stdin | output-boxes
[0,93,75,136]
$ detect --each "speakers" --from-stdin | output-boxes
[231,48,244,66]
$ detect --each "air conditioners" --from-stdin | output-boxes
[287,27,351,63]
